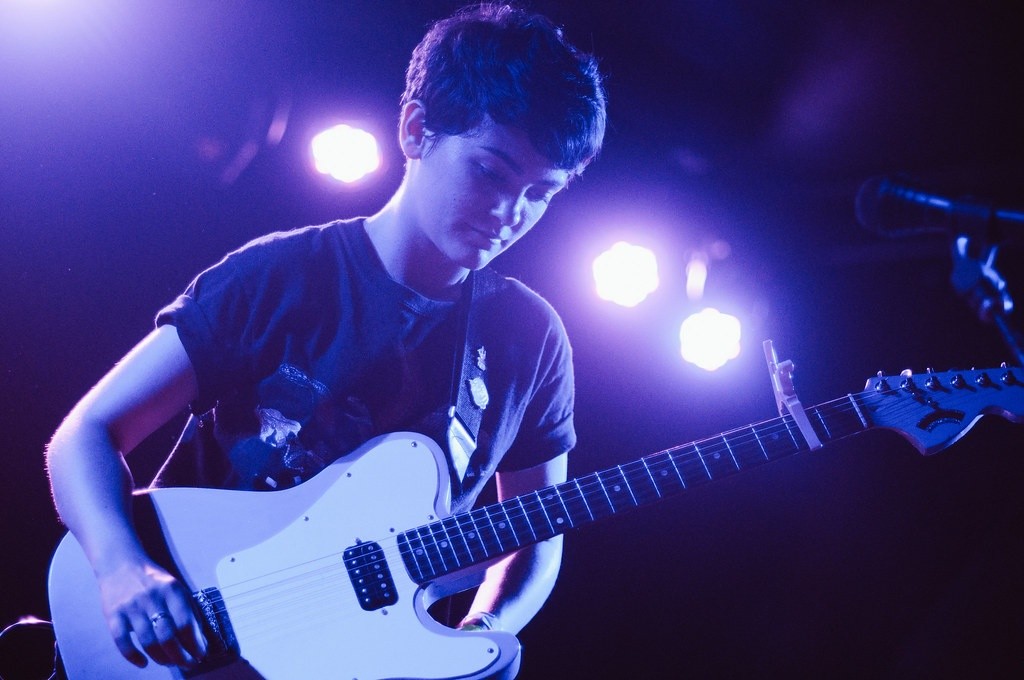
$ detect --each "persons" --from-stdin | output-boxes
[40,3,608,680]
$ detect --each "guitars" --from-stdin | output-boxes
[48,360,1024,680]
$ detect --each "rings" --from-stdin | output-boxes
[150,611,167,624]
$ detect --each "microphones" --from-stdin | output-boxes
[856,178,1024,233]
